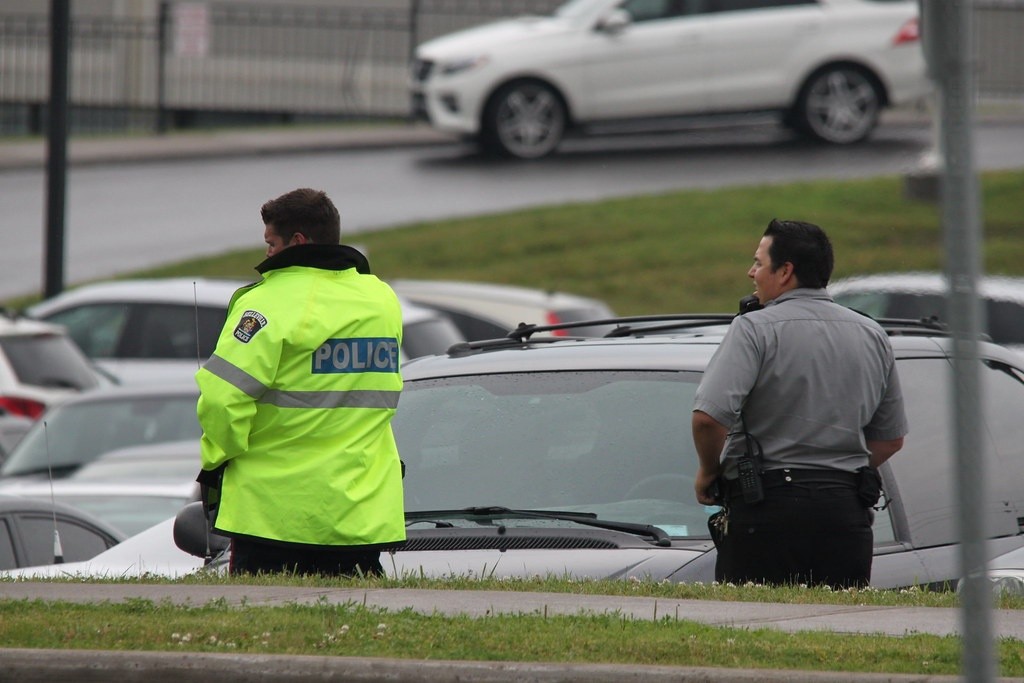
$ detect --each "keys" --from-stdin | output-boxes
[710,514,732,537]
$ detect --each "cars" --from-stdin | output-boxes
[0,274,1024,594]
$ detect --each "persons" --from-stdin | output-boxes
[692,217,908,590]
[197,186,407,581]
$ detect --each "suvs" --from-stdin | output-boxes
[405,0,929,165]
